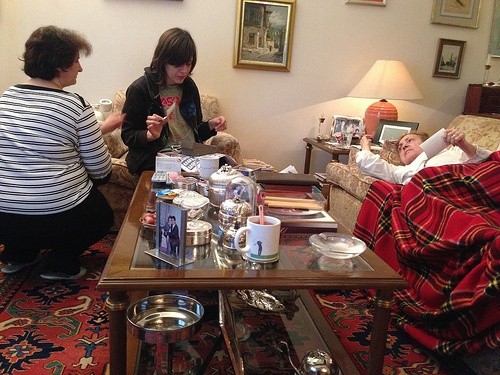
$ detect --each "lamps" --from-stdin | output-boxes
[347,60,422,140]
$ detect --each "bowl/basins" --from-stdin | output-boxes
[139,164,258,251]
[308,230,367,259]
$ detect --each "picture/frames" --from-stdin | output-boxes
[431,0,483,28]
[373,119,419,145]
[432,37,467,79]
[233,0,297,72]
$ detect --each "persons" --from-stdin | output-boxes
[0,26,126,280]
[163,215,180,253]
[356,126,494,186]
[121,28,244,177]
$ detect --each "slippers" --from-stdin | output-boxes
[1,255,42,273]
[40,266,88,280]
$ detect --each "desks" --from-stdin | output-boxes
[302,137,375,210]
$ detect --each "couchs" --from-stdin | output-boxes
[326,114,500,233]
[98,89,242,231]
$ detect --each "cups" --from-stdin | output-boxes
[340,130,354,147]
[233,214,280,263]
[194,156,219,180]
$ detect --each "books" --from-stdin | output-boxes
[254,171,337,229]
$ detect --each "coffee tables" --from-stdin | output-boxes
[96,170,409,374]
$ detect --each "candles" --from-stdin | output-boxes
[487,53,491,66]
